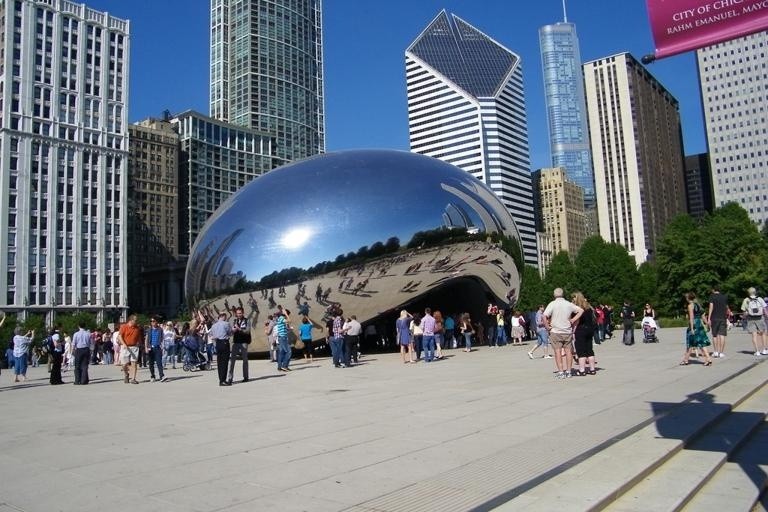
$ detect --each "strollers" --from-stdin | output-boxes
[180,333,212,372]
[641,317,660,344]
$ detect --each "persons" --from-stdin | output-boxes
[679,290,712,366]
[2,309,363,388]
[707,287,730,358]
[692,298,709,357]
[740,286,768,357]
[183,238,520,335]
[619,300,636,345]
[702,296,768,333]
[528,288,612,380]
[397,299,616,364]
[643,302,656,318]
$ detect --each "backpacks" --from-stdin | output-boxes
[747,296,762,316]
[41,335,55,352]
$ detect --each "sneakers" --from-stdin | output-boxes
[762,348,768,355]
[720,352,726,358]
[753,350,761,356]
[123,374,250,386]
[712,351,719,358]
[527,350,597,379]
[276,351,362,372]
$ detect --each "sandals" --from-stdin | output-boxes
[703,360,713,367]
[679,360,689,366]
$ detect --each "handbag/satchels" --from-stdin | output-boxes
[519,318,527,326]
[294,338,305,349]
[396,332,402,345]
